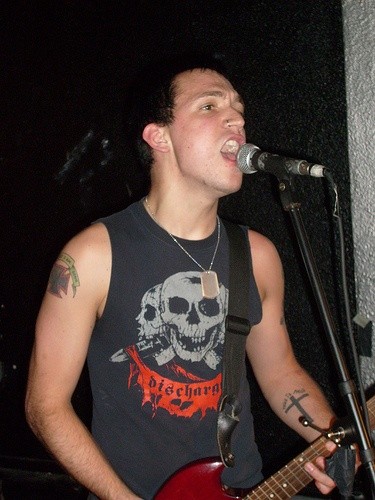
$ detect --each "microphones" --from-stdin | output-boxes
[236,143,329,178]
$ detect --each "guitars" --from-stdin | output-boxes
[154,396,375,500]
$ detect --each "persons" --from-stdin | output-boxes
[25,53,362,500]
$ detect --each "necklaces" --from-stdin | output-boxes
[140,193,230,302]
